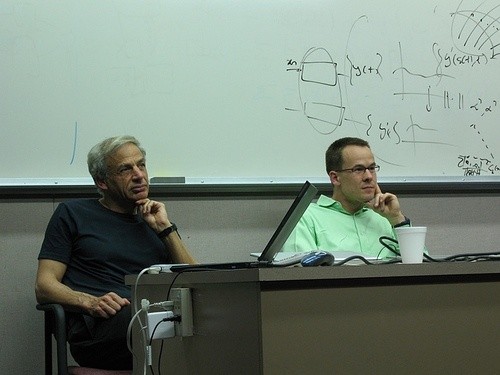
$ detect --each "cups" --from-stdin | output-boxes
[395,226,428,264]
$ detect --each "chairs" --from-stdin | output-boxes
[31,276,100,375]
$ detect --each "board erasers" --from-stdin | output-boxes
[150,177,185,183]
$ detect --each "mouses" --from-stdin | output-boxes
[302,251,334,266]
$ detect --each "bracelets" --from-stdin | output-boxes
[158,221,179,241]
[391,216,411,230]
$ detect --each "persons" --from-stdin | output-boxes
[278,137,415,264]
[36,135,200,371]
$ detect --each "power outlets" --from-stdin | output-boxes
[167,288,194,338]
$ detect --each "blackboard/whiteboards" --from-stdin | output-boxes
[0,0,500,199]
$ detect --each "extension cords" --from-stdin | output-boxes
[170,288,192,337]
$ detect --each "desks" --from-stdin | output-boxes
[116,258,500,375]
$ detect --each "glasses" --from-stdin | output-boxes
[337,165,380,175]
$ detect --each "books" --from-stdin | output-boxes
[249,251,383,266]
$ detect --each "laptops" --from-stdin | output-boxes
[170,181,318,272]
